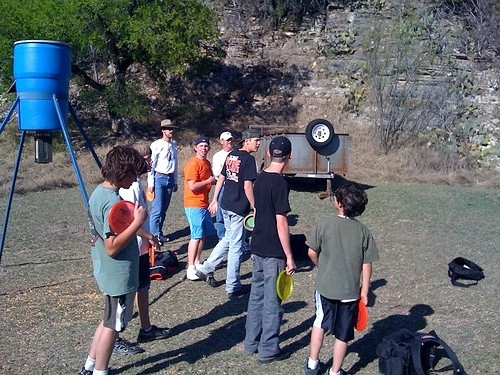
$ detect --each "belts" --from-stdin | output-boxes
[157,172,172,177]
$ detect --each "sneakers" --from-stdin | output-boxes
[187,268,200,281]
[304,355,322,375]
[160,234,169,242]
[111,337,144,354]
[195,269,218,287]
[138,325,172,341]
[227,284,251,298]
[77,364,93,375]
[326,361,343,375]
[196,262,203,269]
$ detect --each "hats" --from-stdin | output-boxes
[156,119,179,133]
[220,131,235,141]
[269,135,292,158]
[238,128,261,146]
[194,136,210,153]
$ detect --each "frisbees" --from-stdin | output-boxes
[145,188,155,202]
[276,270,292,300]
[108,200,135,234]
[244,214,255,231]
[149,245,155,267]
[354,300,368,332]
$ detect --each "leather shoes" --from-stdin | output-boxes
[257,349,291,364]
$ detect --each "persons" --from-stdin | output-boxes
[211,131,251,253]
[113,144,169,354]
[79,146,148,374]
[304,182,379,375]
[147,119,178,246]
[183,136,217,280]
[244,136,297,364]
[195,129,261,294]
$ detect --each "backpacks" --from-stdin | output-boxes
[447,256,485,288]
[375,327,464,375]
